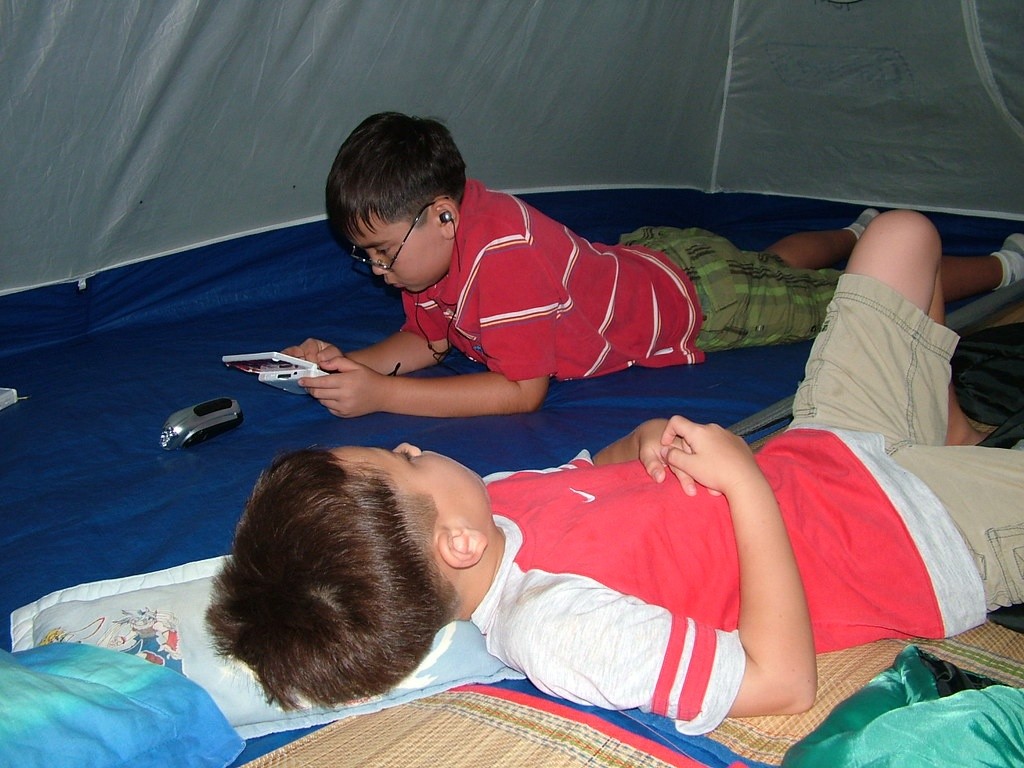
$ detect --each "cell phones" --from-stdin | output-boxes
[221,352,332,395]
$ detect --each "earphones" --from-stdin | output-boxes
[439,210,452,223]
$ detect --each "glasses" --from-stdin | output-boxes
[350,197,452,272]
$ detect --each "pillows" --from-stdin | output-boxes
[9,554,526,739]
[0,642,247,768]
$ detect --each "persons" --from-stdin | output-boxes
[279,111,1024,417]
[200,210,1024,736]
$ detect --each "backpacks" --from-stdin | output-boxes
[945,322,1024,449]
